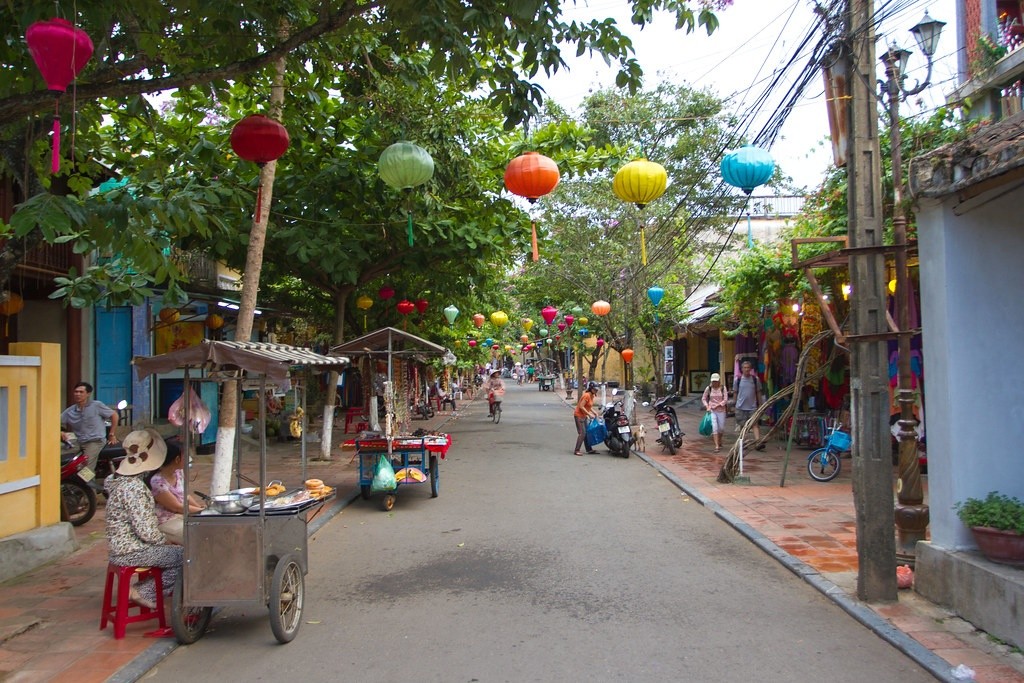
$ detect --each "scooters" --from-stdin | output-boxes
[642,384,685,455]
[417,399,435,421]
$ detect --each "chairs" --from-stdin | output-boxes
[452,387,463,400]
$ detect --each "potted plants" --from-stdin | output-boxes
[950,491,1024,567]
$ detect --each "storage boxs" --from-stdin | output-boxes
[339,442,356,451]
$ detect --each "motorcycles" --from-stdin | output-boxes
[61,400,181,527]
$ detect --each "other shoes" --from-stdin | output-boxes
[575,451,582,455]
[714,448,719,453]
[128,585,136,600]
[453,408,459,411]
[588,450,600,454]
[719,444,723,449]
[487,414,494,417]
[132,591,157,609]
[756,444,765,451]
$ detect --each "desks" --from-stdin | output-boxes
[430,397,441,410]
[344,408,366,434]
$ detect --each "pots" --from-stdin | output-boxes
[193,490,255,513]
[231,480,281,494]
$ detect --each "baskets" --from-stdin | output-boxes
[831,431,851,452]
[493,394,503,402]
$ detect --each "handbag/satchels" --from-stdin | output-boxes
[169,386,211,434]
[699,412,712,435]
[371,455,397,492]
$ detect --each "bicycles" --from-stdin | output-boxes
[806,417,852,482]
[598,388,636,458]
[484,387,504,425]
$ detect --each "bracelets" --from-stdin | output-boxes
[109,433,114,436]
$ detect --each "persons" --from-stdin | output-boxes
[484,369,506,417]
[573,381,600,455]
[452,378,472,400]
[702,373,728,453]
[438,385,459,411]
[103,430,182,608]
[151,436,205,547]
[730,362,766,451]
[513,363,535,385]
[482,361,493,374]
[60,383,118,511]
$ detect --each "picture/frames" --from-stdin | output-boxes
[690,370,711,392]
[663,346,673,385]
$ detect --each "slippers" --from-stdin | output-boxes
[185,614,200,625]
[144,626,174,637]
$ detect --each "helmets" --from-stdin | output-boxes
[589,381,599,388]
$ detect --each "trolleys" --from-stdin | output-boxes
[349,436,439,511]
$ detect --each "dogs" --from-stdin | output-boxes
[630,425,647,452]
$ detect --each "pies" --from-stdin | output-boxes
[250,483,286,496]
[305,478,324,498]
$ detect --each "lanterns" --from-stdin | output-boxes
[26,18,95,174]
[160,307,181,330]
[505,150,561,261]
[0,288,24,337]
[205,314,224,339]
[231,113,289,223]
[376,140,434,248]
[356,285,610,355]
[721,146,774,251]
[613,153,668,267]
[648,284,665,325]
[621,348,634,381]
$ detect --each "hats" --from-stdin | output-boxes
[116,428,167,475]
[710,373,720,382]
[489,370,502,377]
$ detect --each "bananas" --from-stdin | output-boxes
[290,420,301,438]
[290,406,304,420]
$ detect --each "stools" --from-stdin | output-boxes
[357,423,369,431]
[99,561,165,641]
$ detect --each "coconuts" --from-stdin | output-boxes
[266,417,281,436]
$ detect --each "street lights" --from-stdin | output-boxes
[876,8,947,573]
